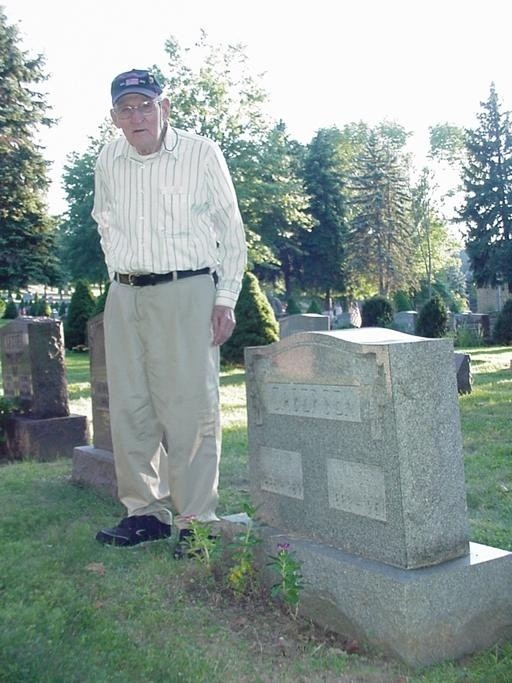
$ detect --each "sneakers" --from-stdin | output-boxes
[96,515,172,548]
[174,529,218,562]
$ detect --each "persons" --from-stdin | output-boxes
[349,302,362,328]
[7,289,53,303]
[90,69,248,561]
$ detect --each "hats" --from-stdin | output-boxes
[111,69,162,106]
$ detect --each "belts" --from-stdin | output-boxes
[114,267,209,286]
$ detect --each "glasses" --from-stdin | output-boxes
[113,100,154,118]
